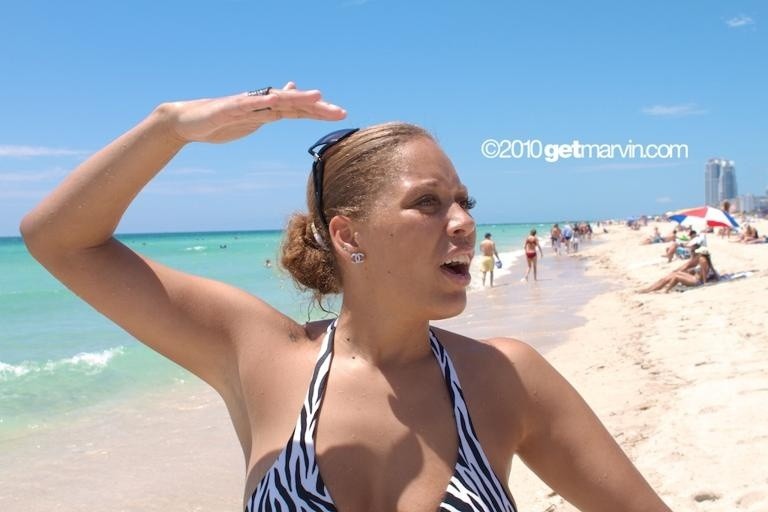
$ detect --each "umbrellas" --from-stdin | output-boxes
[664,204,740,246]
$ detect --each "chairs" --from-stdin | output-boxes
[671,234,710,257]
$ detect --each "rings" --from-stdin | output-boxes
[246,85,272,114]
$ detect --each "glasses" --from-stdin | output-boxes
[308,128,360,242]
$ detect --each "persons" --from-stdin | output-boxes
[478,232,501,288]
[522,228,544,282]
[18,82,675,511]
[653,246,722,294]
[720,201,735,243]
[632,242,704,295]
[626,213,768,260]
[550,220,592,255]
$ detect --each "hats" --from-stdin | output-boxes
[694,246,711,256]
